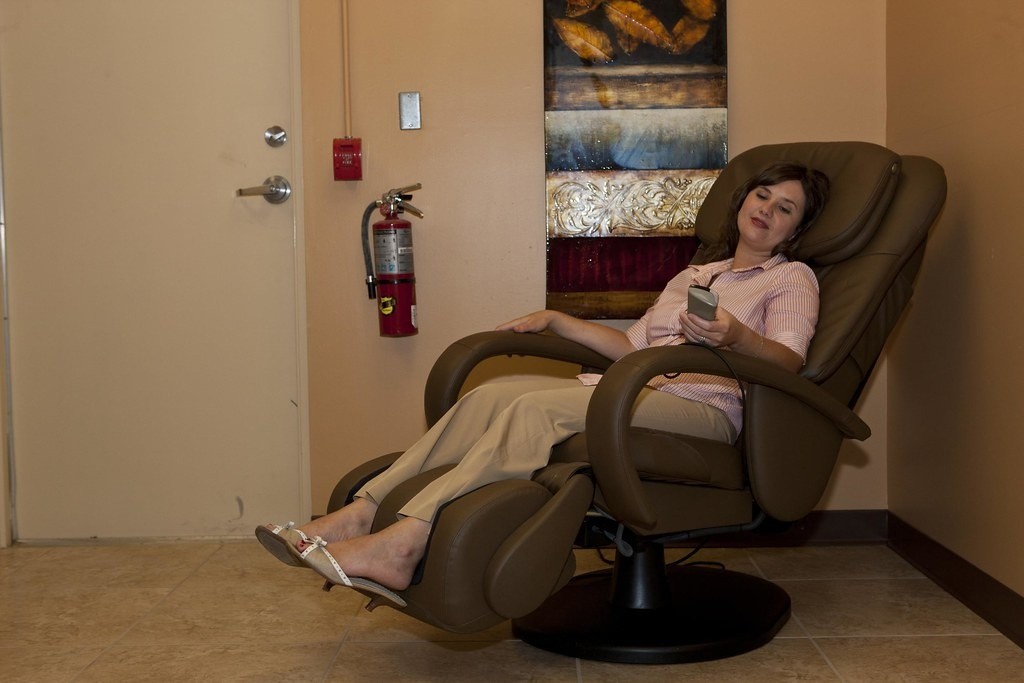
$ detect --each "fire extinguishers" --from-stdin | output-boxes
[363,183,426,340]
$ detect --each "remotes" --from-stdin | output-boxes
[688,284,717,323]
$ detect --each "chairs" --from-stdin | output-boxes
[314,141,948,666]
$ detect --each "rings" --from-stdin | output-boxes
[700,337,705,343]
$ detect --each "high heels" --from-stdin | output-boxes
[287,534,406,612]
[253,521,342,592]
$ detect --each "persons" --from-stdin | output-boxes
[256,159,827,611]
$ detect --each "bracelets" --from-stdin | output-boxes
[755,336,764,359]
